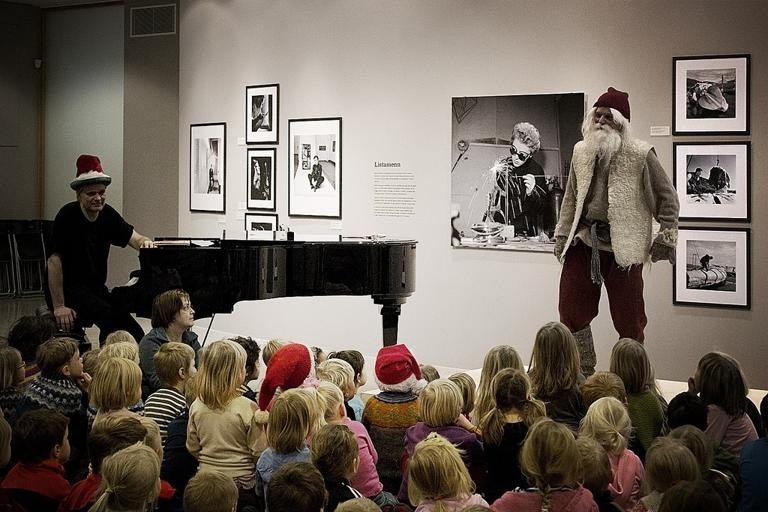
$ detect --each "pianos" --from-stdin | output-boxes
[111,232,418,348]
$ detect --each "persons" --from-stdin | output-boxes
[481,121,551,238]
[251,159,269,199]
[43,153,154,348]
[698,253,714,271]
[0,287,768,511]
[686,167,707,202]
[553,86,680,377]
[208,164,214,189]
[307,155,324,192]
[252,95,265,132]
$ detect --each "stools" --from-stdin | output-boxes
[37,304,91,354]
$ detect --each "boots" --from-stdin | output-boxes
[573,326,597,377]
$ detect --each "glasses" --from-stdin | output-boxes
[509,146,529,161]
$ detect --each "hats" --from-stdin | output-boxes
[70,154,111,189]
[255,344,314,424]
[375,344,427,394]
[592,87,631,122]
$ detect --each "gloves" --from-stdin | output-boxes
[553,236,565,260]
[649,242,677,265]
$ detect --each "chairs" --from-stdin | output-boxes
[0,220,54,299]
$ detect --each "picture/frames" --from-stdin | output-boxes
[672,54,751,135]
[288,117,342,218]
[245,213,278,231]
[190,123,226,212]
[673,226,751,309]
[247,148,276,210]
[673,141,751,222]
[246,84,279,144]
